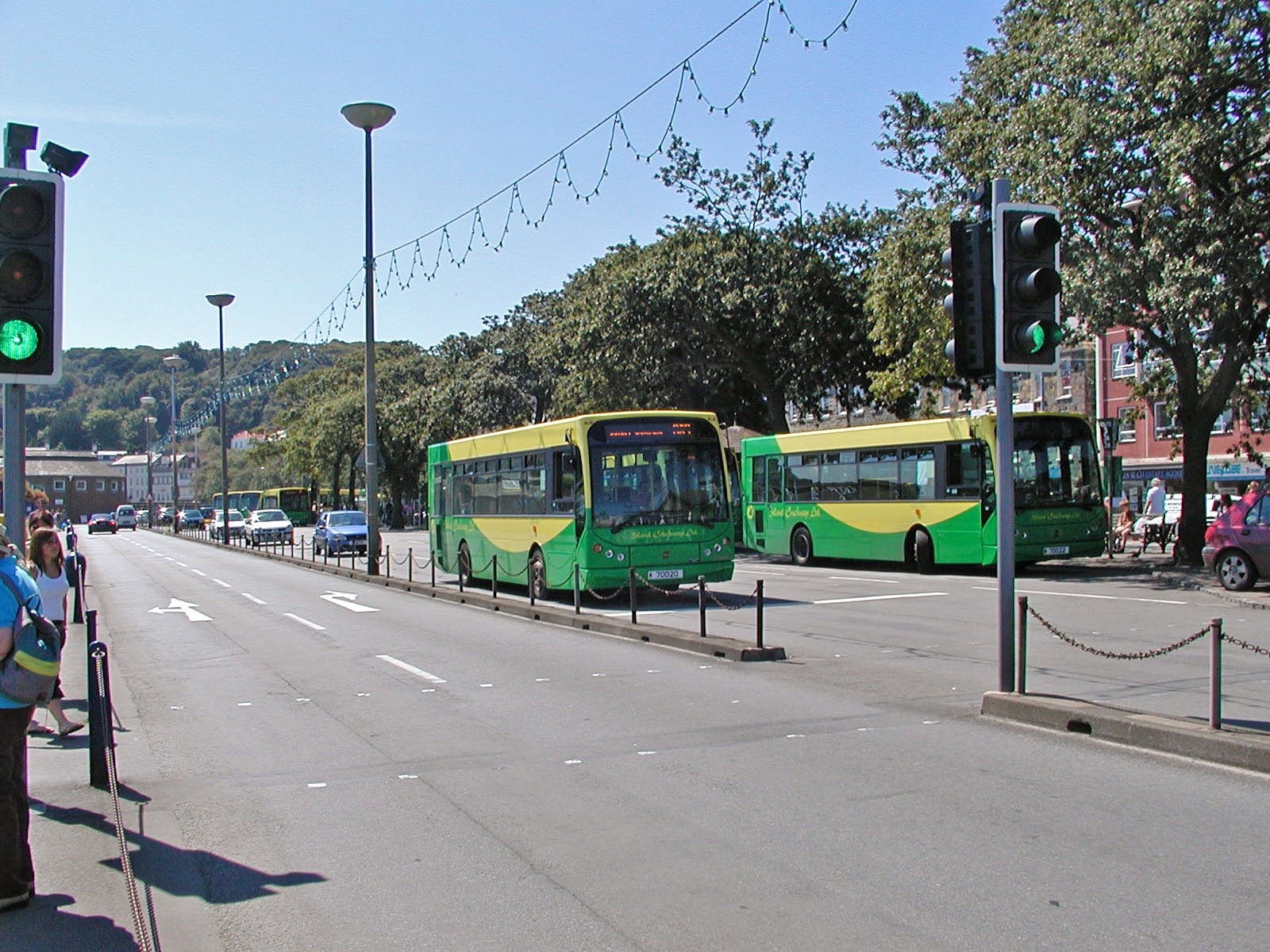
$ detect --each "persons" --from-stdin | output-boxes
[312,495,413,527]
[0,489,85,909]
[1074,477,1259,553]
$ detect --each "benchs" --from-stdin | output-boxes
[1104,513,1167,553]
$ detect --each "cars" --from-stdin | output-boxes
[1202,488,1270,592]
[89,513,116,533]
[136,507,212,530]
[313,511,381,556]
[208,509,247,538]
[244,510,295,546]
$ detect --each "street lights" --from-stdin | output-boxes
[341,99,396,574]
[139,396,155,527]
[163,354,183,531]
[206,292,236,544]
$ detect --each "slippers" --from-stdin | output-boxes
[58,720,85,736]
[28,719,55,733]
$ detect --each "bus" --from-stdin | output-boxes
[740,413,1106,573]
[211,486,389,524]
[426,410,745,601]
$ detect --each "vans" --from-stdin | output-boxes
[116,504,137,532]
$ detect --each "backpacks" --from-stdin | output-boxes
[1,570,61,705]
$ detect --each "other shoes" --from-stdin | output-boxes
[1115,546,1125,553]
[12,873,35,898]
[0,876,28,911]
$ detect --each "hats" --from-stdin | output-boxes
[1118,500,1129,505]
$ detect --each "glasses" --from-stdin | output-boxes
[45,540,59,546]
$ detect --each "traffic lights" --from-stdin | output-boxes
[0,169,63,384]
[993,205,1064,379]
[941,221,993,379]
